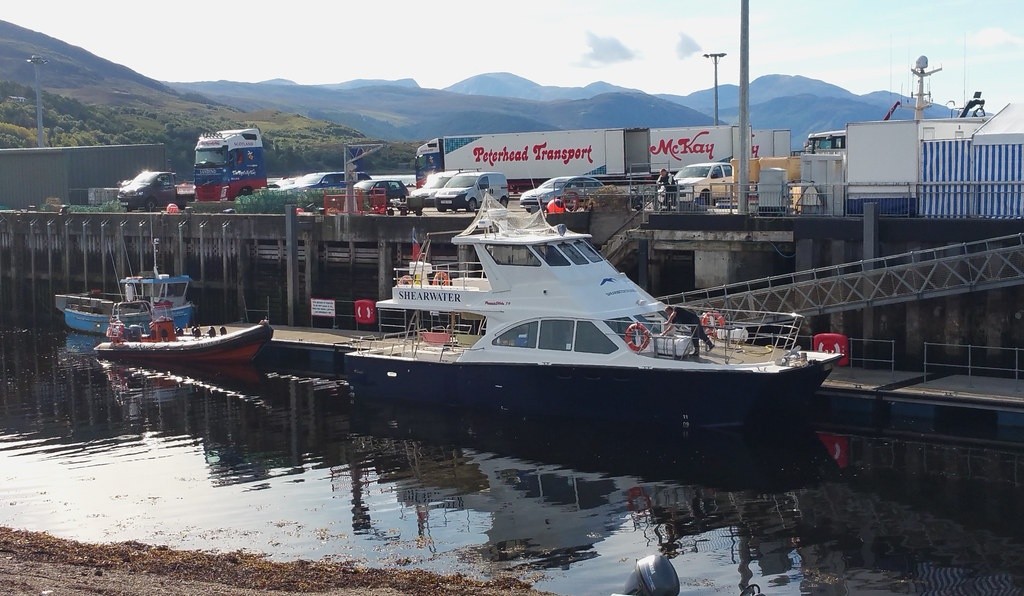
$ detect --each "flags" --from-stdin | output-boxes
[412,227,423,262]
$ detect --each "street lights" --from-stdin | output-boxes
[704,53,727,126]
[26,53,50,147]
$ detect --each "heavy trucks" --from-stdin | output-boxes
[415,128,650,193]
[802,130,845,155]
[651,125,791,176]
[193,128,280,201]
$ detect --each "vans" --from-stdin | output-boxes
[284,172,372,190]
[412,171,465,206]
[435,172,508,211]
[657,163,731,204]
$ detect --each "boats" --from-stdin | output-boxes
[94,301,274,363]
[345,168,846,425]
[64,209,193,335]
[105,364,266,407]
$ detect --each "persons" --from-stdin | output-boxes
[656,168,677,209]
[661,306,715,357]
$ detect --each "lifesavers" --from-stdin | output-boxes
[399,276,414,285]
[701,312,725,337]
[433,272,450,286]
[625,324,651,351]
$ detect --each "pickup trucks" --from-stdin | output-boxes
[118,172,196,211]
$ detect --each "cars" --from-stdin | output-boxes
[353,181,409,202]
[519,176,606,213]
